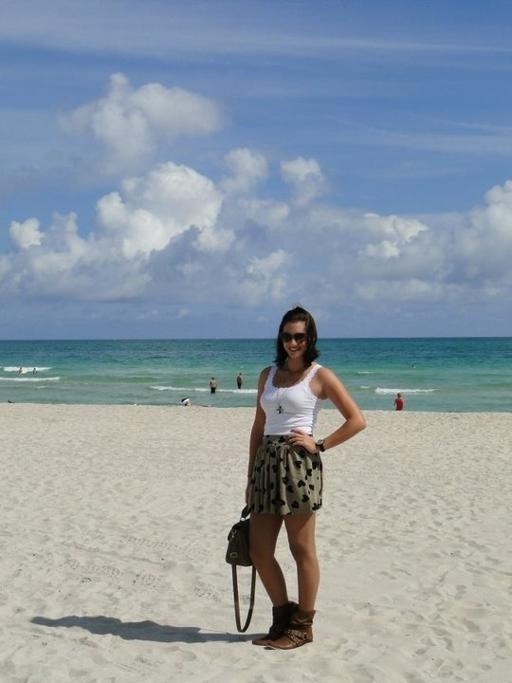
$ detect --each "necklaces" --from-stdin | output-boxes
[274,375,295,413]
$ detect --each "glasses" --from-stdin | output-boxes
[279,332,305,342]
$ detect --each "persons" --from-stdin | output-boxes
[209,376,217,393]
[392,393,405,409]
[181,397,192,406]
[245,305,369,649]
[236,372,243,388]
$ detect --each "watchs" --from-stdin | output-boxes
[315,439,324,453]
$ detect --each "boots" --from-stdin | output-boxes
[252,602,316,650]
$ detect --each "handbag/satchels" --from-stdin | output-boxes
[226,506,253,566]
